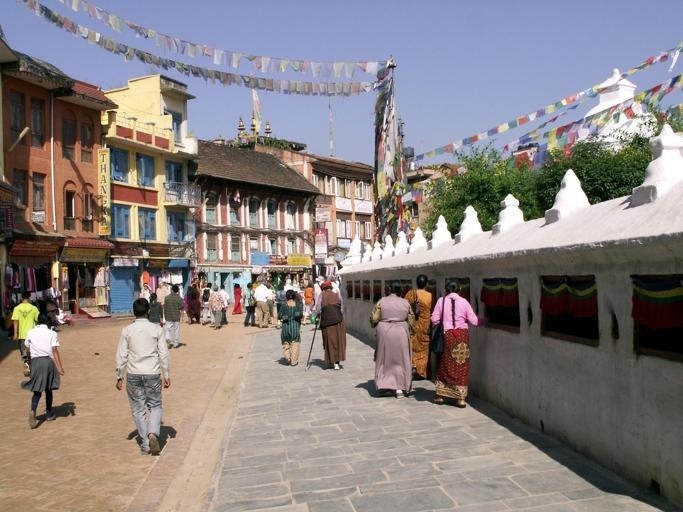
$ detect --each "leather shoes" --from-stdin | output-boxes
[433,397,466,408]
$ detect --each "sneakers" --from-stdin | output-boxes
[149,434,160,455]
[396,391,415,398]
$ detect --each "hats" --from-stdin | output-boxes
[316,275,324,281]
[321,281,332,290]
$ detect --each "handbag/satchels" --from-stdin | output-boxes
[371,304,381,328]
[410,302,420,321]
[431,324,443,355]
[248,295,257,307]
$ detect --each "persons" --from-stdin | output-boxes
[141,274,346,370]
[404,275,433,379]
[11,291,40,376]
[113,297,170,456]
[370,283,417,400]
[20,312,63,429]
[431,281,478,408]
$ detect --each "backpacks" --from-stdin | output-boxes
[203,289,210,302]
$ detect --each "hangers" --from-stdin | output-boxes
[6,258,48,271]
